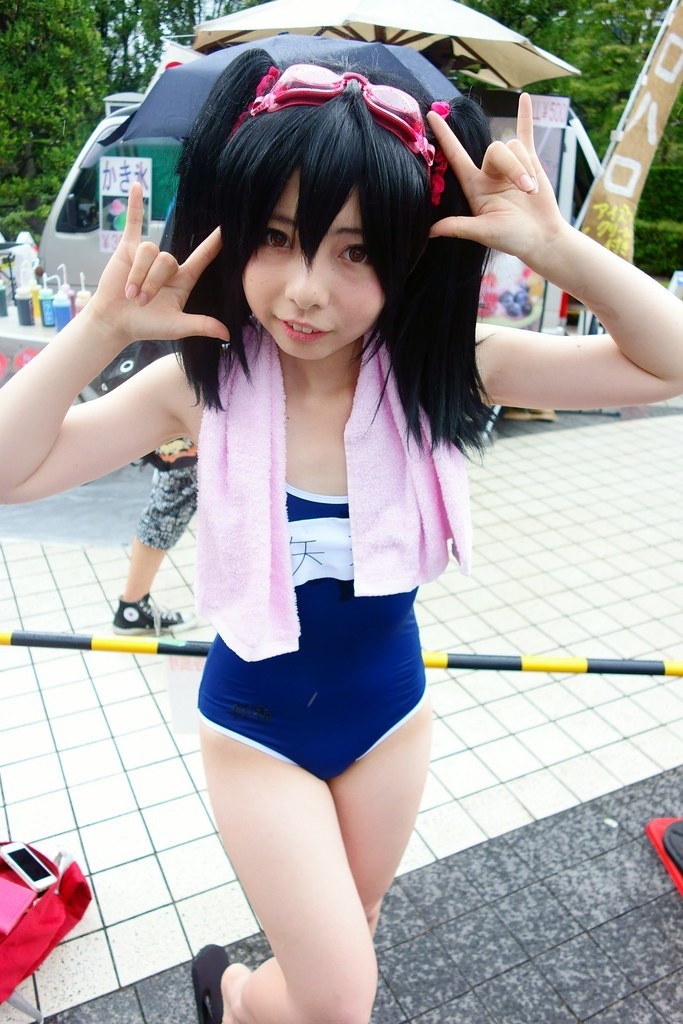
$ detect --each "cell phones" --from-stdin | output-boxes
[0,841,58,893]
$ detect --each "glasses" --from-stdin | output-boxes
[226,62,443,207]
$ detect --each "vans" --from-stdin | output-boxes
[32,90,186,296]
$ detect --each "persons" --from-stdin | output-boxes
[112,200,194,634]
[0,48,683,1023]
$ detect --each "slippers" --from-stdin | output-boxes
[191,944,230,1024]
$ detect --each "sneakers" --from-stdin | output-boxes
[112,595,197,637]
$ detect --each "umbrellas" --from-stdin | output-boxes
[193,0,582,93]
[121,34,462,143]
